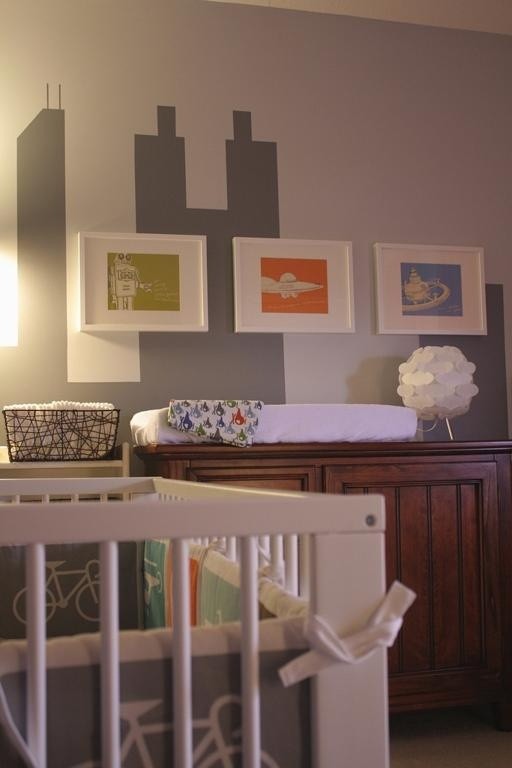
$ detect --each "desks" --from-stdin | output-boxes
[0,442,129,501]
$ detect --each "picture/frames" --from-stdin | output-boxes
[78,231,209,333]
[232,236,356,334]
[374,242,488,336]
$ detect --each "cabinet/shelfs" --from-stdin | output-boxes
[135,440,512,726]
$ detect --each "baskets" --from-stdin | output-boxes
[2,408,120,462]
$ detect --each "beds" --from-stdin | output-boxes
[0,475,392,768]
